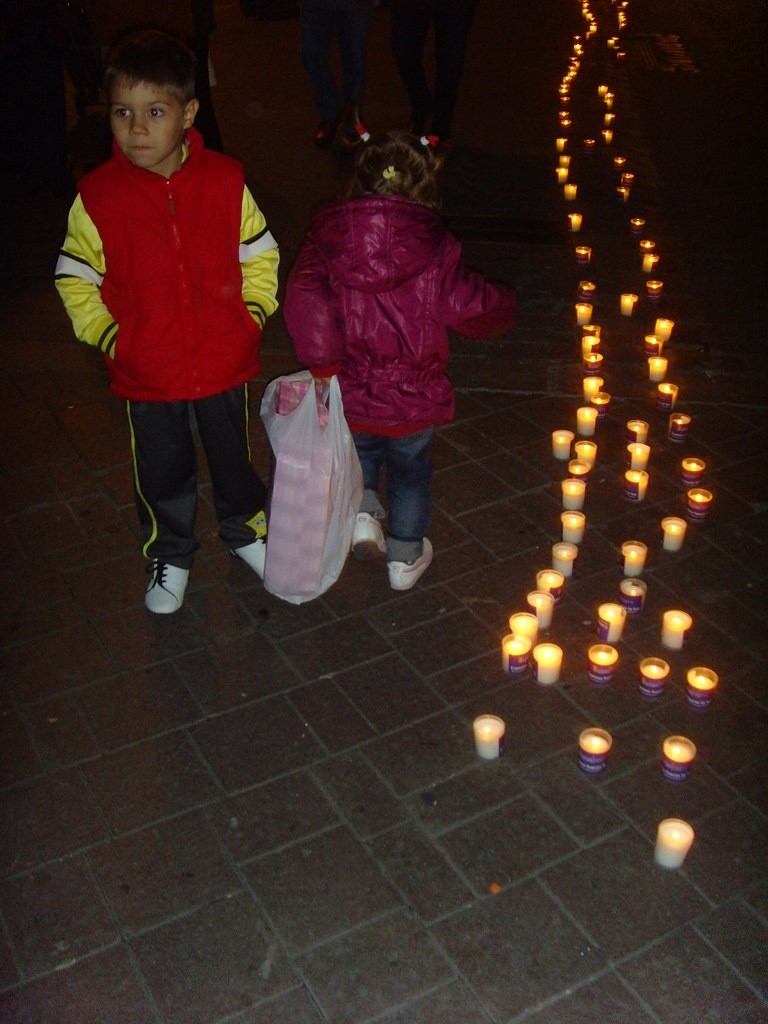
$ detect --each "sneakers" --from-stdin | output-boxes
[387,536,433,591]
[229,538,266,580]
[144,554,189,614]
[350,512,387,560]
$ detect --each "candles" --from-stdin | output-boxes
[465,0,714,867]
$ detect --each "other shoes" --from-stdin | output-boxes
[314,117,338,145]
[343,100,363,136]
[431,120,450,140]
[409,99,433,134]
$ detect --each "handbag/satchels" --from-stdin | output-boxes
[260,370,364,606]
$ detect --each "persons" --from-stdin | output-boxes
[296,0,382,145]
[53,0,226,152]
[284,117,516,591]
[390,0,478,149]
[51,30,281,617]
